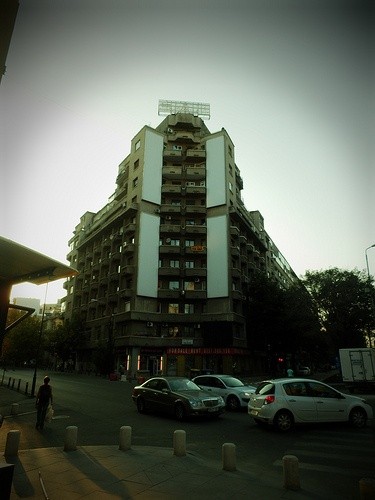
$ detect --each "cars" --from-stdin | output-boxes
[131,377,225,420]
[299,367,312,378]
[191,375,257,411]
[248,378,373,433]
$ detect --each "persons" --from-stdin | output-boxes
[36,376,53,429]
[287,367,293,377]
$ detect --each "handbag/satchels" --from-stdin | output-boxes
[47,406,54,418]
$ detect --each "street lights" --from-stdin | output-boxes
[364,244,375,277]
[91,298,114,373]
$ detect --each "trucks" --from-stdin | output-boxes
[339,346,375,391]
